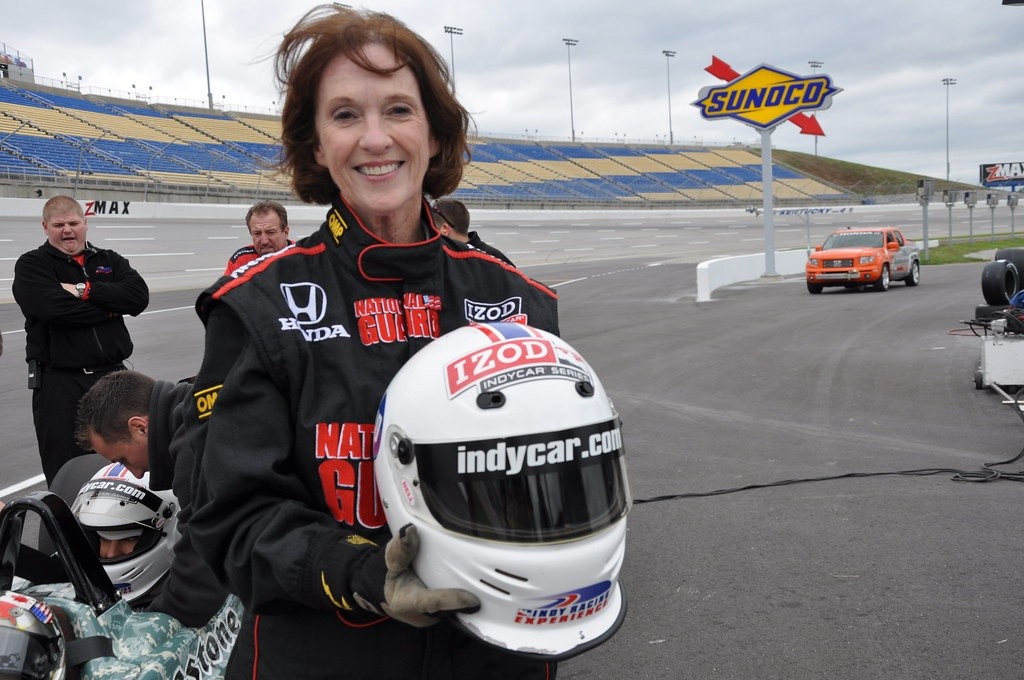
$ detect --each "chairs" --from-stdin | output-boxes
[39,455,111,557]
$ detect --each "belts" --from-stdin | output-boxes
[45,363,124,375]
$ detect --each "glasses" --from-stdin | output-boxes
[429,200,454,228]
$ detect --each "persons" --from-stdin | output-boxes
[430,198,516,267]
[188,9,561,680]
[225,201,293,275]
[12,196,150,491]
[74,370,231,627]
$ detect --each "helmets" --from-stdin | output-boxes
[0,591,66,680]
[70,463,182,604]
[372,323,633,662]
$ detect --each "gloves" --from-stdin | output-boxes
[300,524,481,629]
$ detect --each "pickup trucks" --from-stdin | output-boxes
[811,230,922,291]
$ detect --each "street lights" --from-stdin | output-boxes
[942,77,955,181]
[444,26,463,101]
[563,37,579,141]
[808,60,822,155]
[663,47,676,144]
